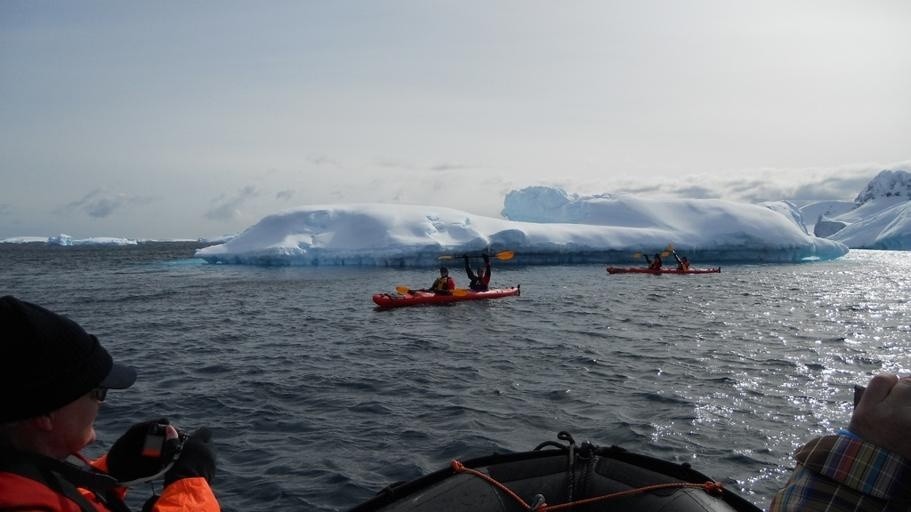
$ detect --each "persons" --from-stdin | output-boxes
[0,296,221,512]
[643,253,662,271]
[462,253,491,292]
[672,249,689,270]
[768,373,911,512]
[423,267,455,295]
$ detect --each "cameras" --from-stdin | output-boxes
[137,423,192,465]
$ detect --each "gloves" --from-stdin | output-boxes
[107,417,217,486]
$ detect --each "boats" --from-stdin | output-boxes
[605,264,723,275]
[332,435,765,512]
[371,281,521,307]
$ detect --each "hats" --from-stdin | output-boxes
[1,295,138,425]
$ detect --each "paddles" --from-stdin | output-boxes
[667,244,687,272]
[395,286,470,297]
[438,251,513,261]
[633,252,670,257]
